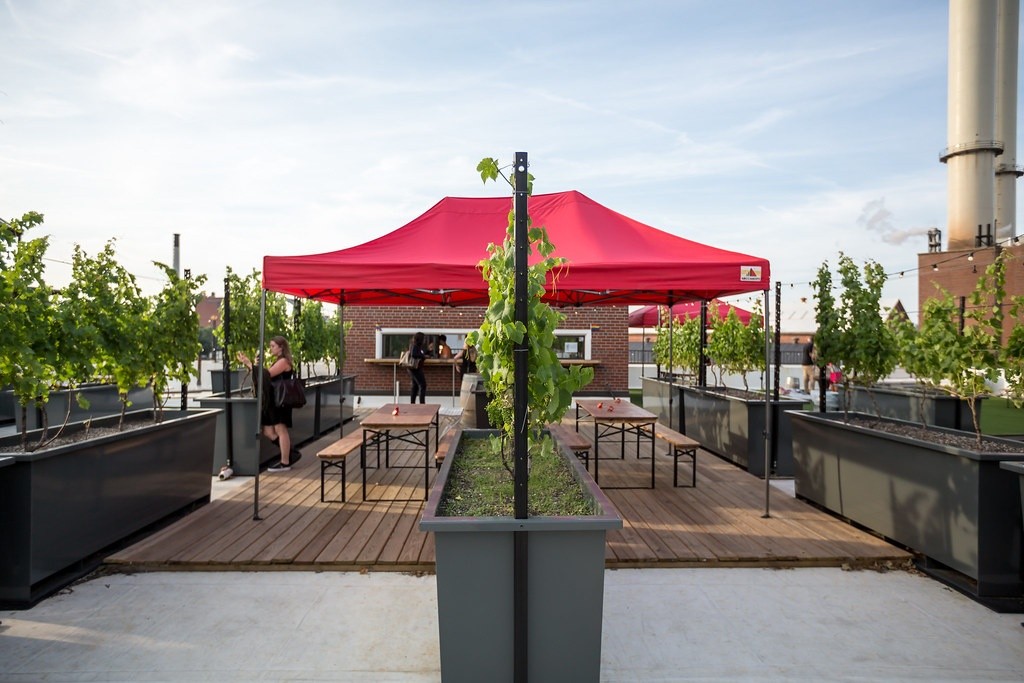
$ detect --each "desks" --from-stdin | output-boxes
[358,403,441,502]
[574,399,659,490]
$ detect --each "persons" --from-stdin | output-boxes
[802,337,816,394]
[236,336,302,471]
[405,332,430,404]
[438,335,453,357]
[454,333,479,380]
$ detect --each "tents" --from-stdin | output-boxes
[254,188,770,519]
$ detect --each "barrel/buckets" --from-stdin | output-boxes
[786,376,799,388]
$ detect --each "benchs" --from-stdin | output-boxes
[634,421,700,487]
[316,425,384,503]
[435,428,458,475]
[542,422,592,473]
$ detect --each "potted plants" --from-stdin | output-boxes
[0,158,624,682]
[650,252,1024,613]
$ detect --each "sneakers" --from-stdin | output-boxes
[267,461,291,472]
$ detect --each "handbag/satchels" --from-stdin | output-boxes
[271,359,306,408]
[399,342,420,370]
